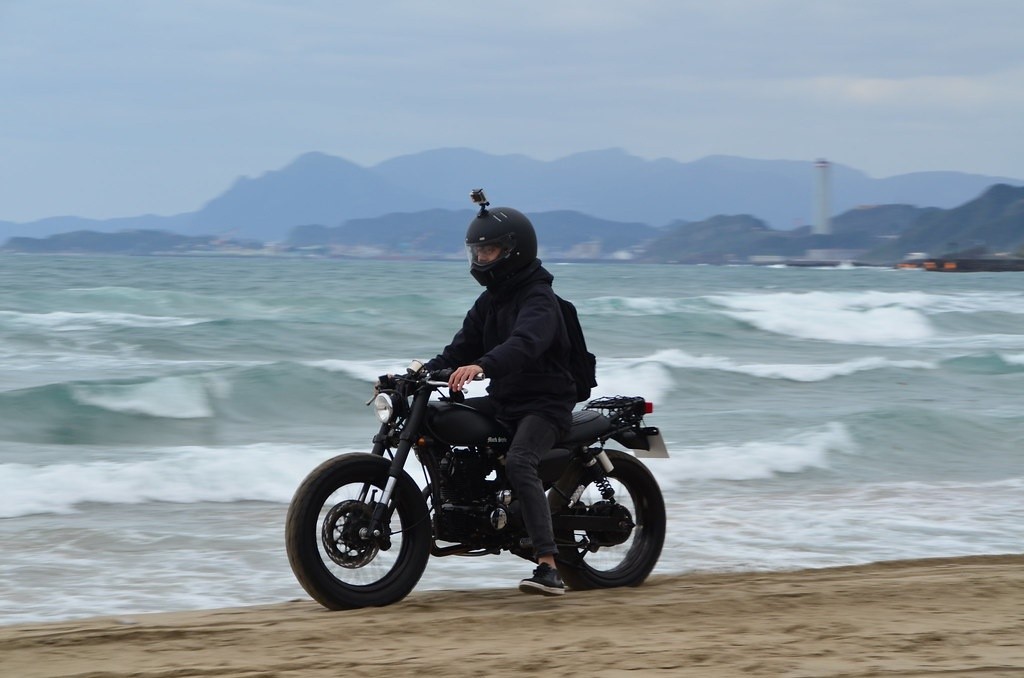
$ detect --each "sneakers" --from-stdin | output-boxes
[520,562,566,595]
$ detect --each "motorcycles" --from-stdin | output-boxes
[286,360,669,612]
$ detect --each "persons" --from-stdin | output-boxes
[373,206,580,598]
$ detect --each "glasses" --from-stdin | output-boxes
[473,246,496,255]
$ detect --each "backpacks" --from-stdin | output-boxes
[480,292,597,389]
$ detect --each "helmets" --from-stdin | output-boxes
[466,188,538,286]
